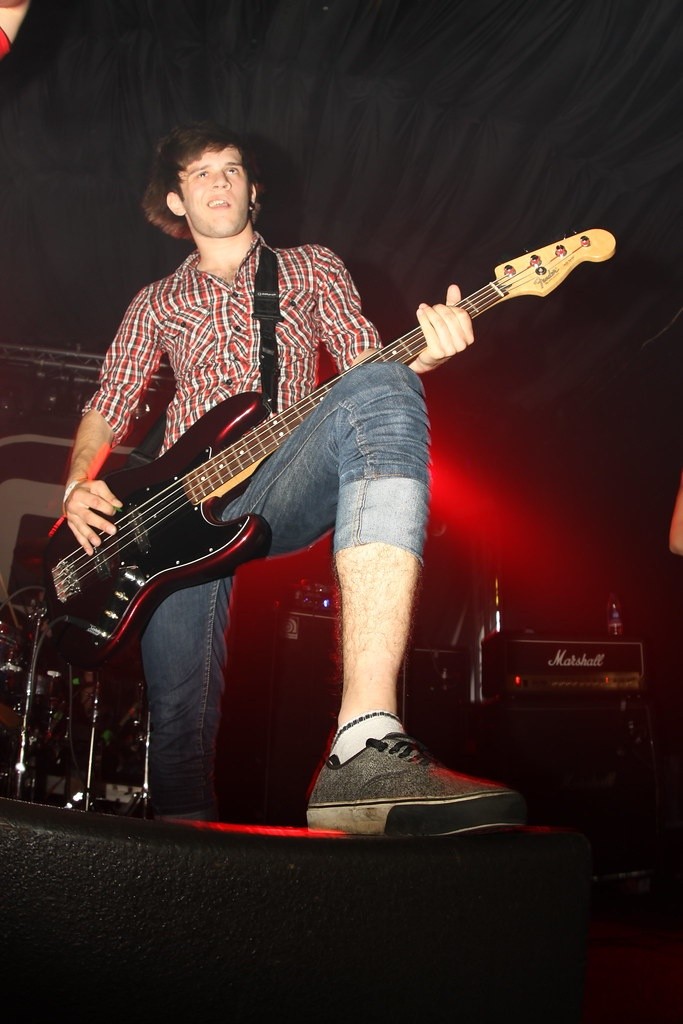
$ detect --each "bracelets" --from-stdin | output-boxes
[48,478,88,539]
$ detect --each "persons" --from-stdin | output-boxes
[60,117,526,839]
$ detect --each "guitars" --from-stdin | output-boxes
[41,224,619,669]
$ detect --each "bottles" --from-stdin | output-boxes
[607,591,623,636]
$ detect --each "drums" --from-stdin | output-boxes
[0,621,29,706]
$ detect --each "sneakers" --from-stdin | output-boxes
[307,733,527,837]
